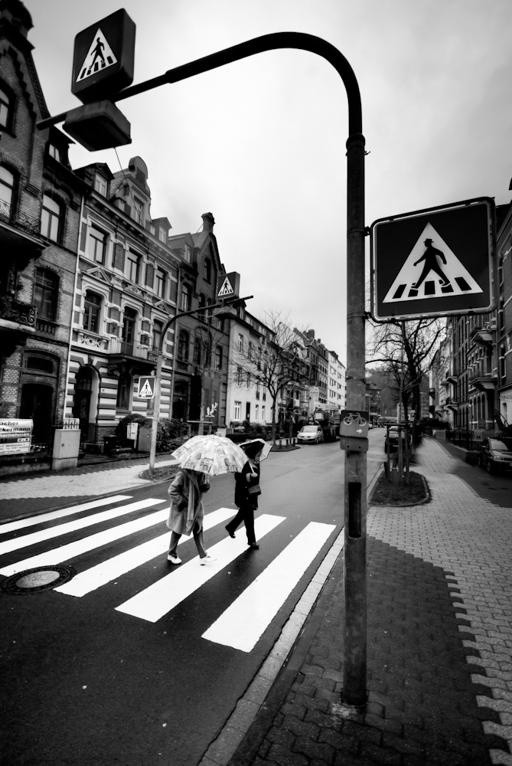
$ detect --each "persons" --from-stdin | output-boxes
[167,469,211,564]
[226,445,261,549]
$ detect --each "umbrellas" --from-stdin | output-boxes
[171,435,249,483]
[237,438,273,461]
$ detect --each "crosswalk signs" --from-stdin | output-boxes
[216,271,240,301]
[70,5,138,101]
[369,192,500,323]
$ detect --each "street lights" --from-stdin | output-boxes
[31,31,373,723]
[147,293,255,476]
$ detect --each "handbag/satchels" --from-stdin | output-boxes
[247,485,261,497]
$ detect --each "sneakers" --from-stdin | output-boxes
[248,543,259,549]
[167,556,181,564]
[201,556,215,566]
[225,526,235,538]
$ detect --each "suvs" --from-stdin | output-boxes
[382,425,412,451]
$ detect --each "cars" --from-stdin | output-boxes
[297,424,324,445]
[479,434,512,477]
